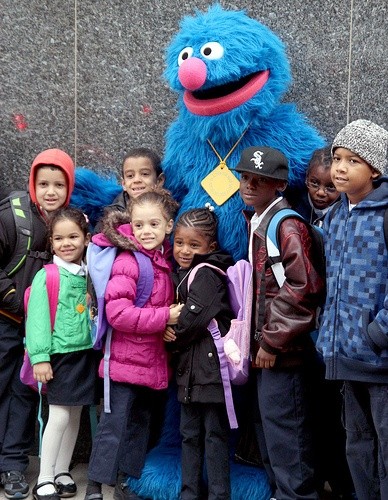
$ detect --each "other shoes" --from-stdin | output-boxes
[84,493,103,500]
[113,484,142,500]
[32,481,61,500]
[73,437,92,463]
[54,472,77,497]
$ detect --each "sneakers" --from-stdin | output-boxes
[0,470,29,499]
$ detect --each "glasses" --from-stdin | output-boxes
[305,178,336,195]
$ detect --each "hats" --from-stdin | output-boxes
[331,119,388,174]
[228,147,288,180]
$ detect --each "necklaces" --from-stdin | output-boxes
[198,123,250,206]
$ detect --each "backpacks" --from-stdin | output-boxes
[86,242,154,350]
[254,208,327,341]
[20,263,59,394]
[187,259,253,384]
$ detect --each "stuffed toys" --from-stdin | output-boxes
[28,3,332,499]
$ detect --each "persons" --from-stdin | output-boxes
[230,146,326,500]
[20,207,98,500]
[293,146,343,228]
[163,202,237,500]
[84,189,184,500]
[95,146,181,236]
[316,118,388,500]
[0,148,91,500]
